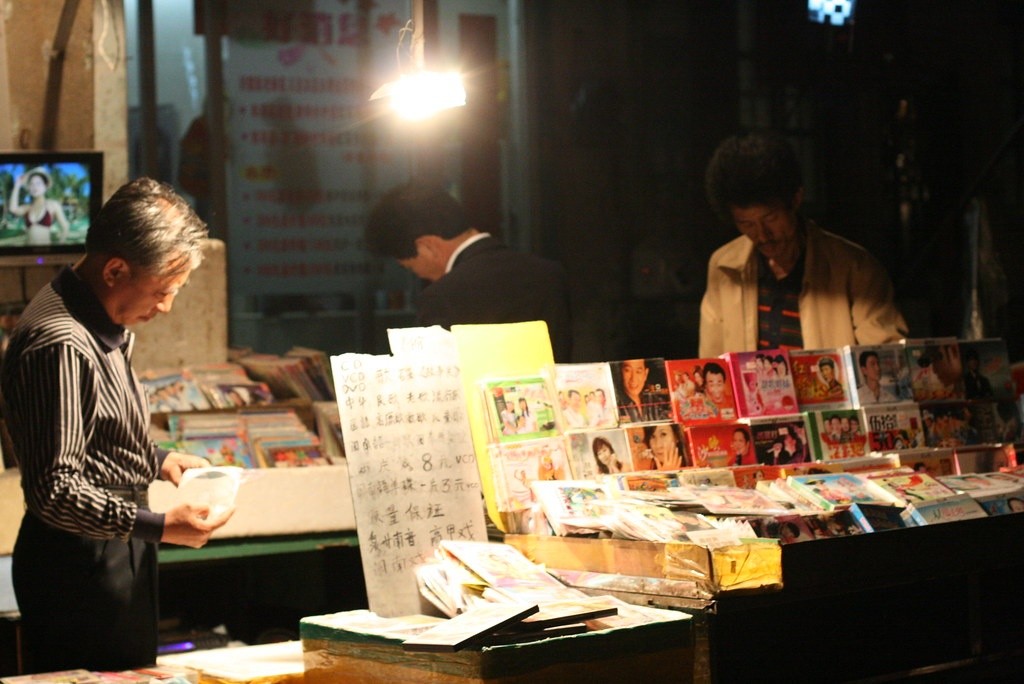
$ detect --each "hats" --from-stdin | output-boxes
[22,165,54,190]
[818,357,834,367]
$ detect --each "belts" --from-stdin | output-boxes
[104,488,149,507]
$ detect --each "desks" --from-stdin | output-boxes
[300,513,1024,684]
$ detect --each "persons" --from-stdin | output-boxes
[0,177,238,674]
[9,166,69,246]
[366,178,577,363]
[180,94,232,241]
[699,133,909,358]
[501,346,1024,541]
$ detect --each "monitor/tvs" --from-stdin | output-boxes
[0,151,104,266]
